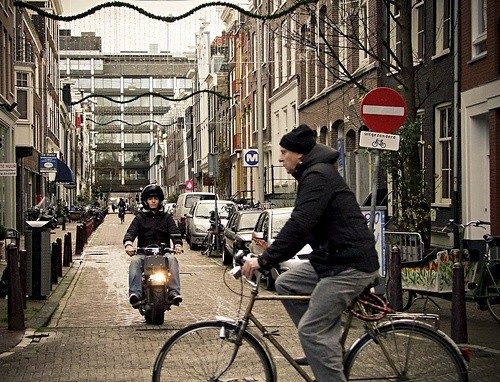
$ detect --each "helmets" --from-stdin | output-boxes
[141,184,164,208]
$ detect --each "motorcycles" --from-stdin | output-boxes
[125,243,183,324]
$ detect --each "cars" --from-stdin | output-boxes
[184,200,237,250]
[248,207,313,291]
[220,211,263,265]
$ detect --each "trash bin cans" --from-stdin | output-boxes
[24,220,50,300]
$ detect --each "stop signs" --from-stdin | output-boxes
[361,87,406,134]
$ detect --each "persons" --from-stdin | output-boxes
[118,198,126,224]
[93,199,101,208]
[123,185,183,304]
[241,124,380,381]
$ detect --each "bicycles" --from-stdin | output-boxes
[152,249,470,382]
[201,221,217,257]
[442,219,500,323]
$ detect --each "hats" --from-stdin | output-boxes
[280,124,315,153]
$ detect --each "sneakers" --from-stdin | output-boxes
[129,294,139,309]
[169,294,182,301]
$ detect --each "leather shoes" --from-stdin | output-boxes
[294,357,309,364]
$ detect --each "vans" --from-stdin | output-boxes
[173,192,219,238]
